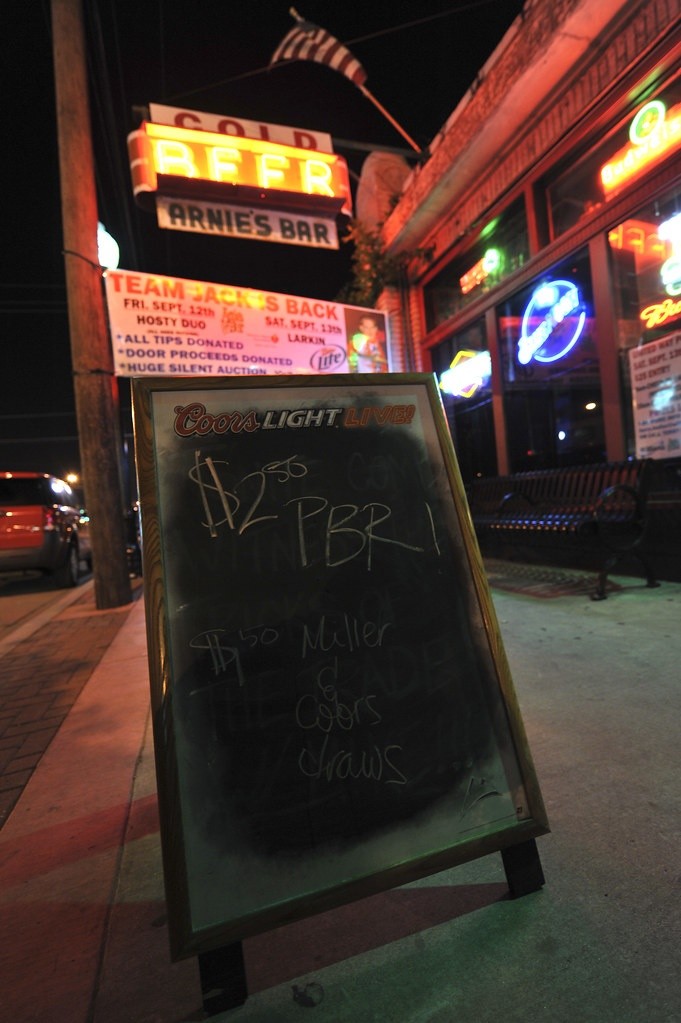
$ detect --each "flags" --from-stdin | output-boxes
[271,16,366,85]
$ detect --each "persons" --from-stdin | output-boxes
[352,316,388,373]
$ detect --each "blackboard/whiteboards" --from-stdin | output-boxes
[122,370,558,969]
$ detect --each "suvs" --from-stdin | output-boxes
[0,471,92,588]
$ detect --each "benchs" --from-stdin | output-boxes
[467,457,661,601]
